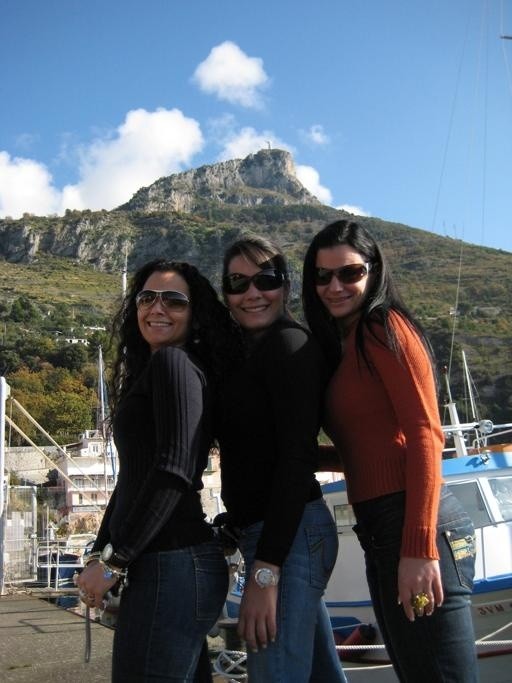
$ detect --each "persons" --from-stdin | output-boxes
[294,219,485,681]
[70,255,250,682]
[205,233,353,681]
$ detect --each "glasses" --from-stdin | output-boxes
[310,262,371,286]
[222,269,286,295]
[135,289,193,312]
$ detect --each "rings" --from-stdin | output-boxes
[410,591,430,610]
[85,596,94,602]
[79,589,89,598]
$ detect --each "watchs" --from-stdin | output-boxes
[251,566,281,589]
[100,543,133,568]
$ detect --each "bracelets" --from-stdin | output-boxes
[84,550,101,568]
[99,560,128,584]
